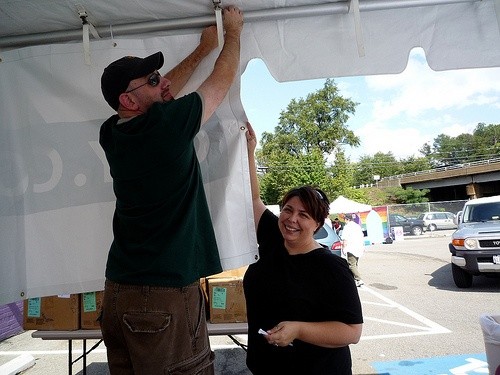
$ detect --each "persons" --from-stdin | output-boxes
[98,6,244,375]
[243,121,363,375]
[332,213,366,287]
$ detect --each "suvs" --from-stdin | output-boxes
[448,195,500,289]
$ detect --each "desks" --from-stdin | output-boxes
[31,322,249,375]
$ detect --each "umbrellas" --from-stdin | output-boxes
[328,195,371,215]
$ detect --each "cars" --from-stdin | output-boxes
[313,222,342,258]
[389,213,428,236]
[417,212,458,232]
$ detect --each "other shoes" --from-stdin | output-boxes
[355,279,363,286]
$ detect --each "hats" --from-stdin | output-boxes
[101,50,164,110]
[344,212,351,219]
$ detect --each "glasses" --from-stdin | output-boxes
[311,187,324,199]
[126,70,161,92]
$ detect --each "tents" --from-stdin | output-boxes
[0,0,500,308]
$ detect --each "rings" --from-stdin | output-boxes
[274,343,278,348]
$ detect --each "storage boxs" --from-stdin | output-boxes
[23,293,80,331]
[207,278,248,324]
[80,291,104,329]
